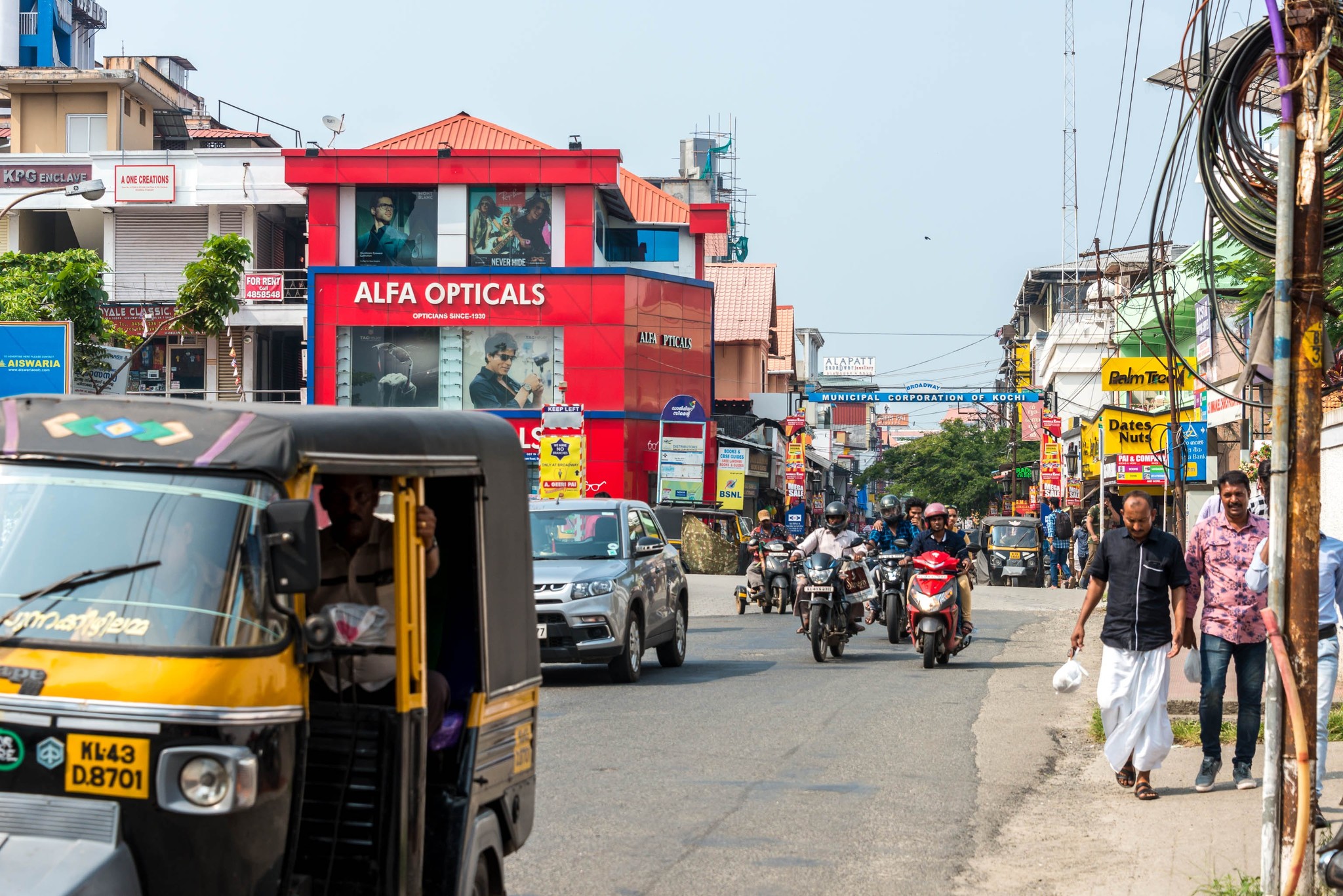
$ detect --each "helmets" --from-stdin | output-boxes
[924,503,948,530]
[1081,516,1087,525]
[825,501,848,533]
[879,494,901,523]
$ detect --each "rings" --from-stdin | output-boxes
[419,521,428,529]
[857,555,860,558]
[967,559,971,562]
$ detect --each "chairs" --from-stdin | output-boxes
[583,516,619,542]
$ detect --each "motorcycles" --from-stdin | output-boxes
[978,515,1045,589]
[893,537,981,670]
[862,539,911,644]
[734,532,804,616]
[651,499,754,575]
[782,536,864,663]
[0,388,542,896]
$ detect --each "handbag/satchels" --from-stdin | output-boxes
[1184,643,1202,683]
[1052,647,1089,695]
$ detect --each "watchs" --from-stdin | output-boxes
[423,538,438,555]
[522,383,532,394]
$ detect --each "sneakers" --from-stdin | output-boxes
[1196,756,1223,792]
[1232,762,1257,790]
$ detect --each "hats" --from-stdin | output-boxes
[758,510,770,521]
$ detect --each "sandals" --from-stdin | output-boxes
[1134,782,1159,800]
[865,600,876,625]
[1115,754,1136,788]
[796,622,809,634]
[851,622,865,631]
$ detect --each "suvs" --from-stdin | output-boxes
[526,499,689,680]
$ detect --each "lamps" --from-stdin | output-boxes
[437,142,453,158]
[569,135,582,151]
[304,141,324,157]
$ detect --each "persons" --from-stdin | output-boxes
[1046,497,1077,589]
[899,502,973,634]
[1069,490,1189,801]
[747,509,797,600]
[1064,516,1087,588]
[1187,459,1343,821]
[865,494,928,625]
[357,192,414,268]
[468,196,551,270]
[1079,492,1120,589]
[468,333,547,409]
[789,501,868,634]
[308,472,463,783]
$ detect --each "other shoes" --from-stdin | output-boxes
[1048,576,1075,588]
[1314,803,1328,827]
[752,590,766,600]
[962,623,973,633]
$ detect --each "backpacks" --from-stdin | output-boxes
[1053,510,1073,539]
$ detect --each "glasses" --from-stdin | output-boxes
[495,353,516,361]
[479,202,545,220]
[375,203,396,210]
[944,505,958,509]
[372,343,413,394]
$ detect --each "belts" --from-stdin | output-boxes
[1318,624,1337,640]
